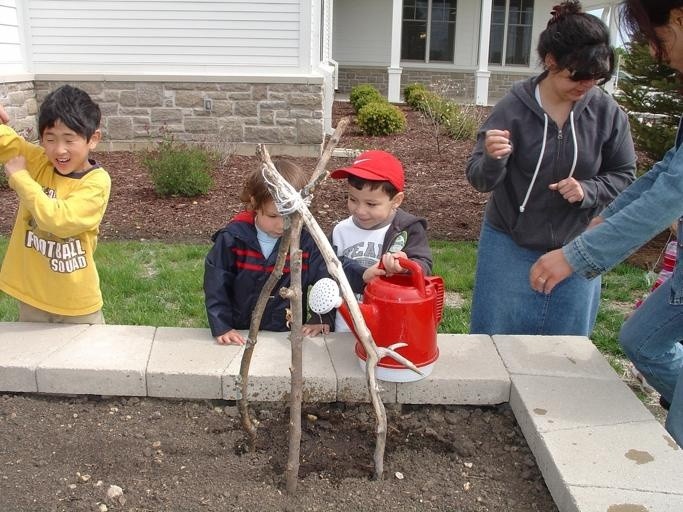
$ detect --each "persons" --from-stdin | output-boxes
[0,84,112,324]
[464,2,637,338]
[527,2,683,449]
[202,156,394,345]
[300,148,434,338]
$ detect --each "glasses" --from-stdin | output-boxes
[558,55,605,82]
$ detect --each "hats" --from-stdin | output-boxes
[331,149,405,192]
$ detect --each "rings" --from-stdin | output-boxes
[537,276,546,284]
